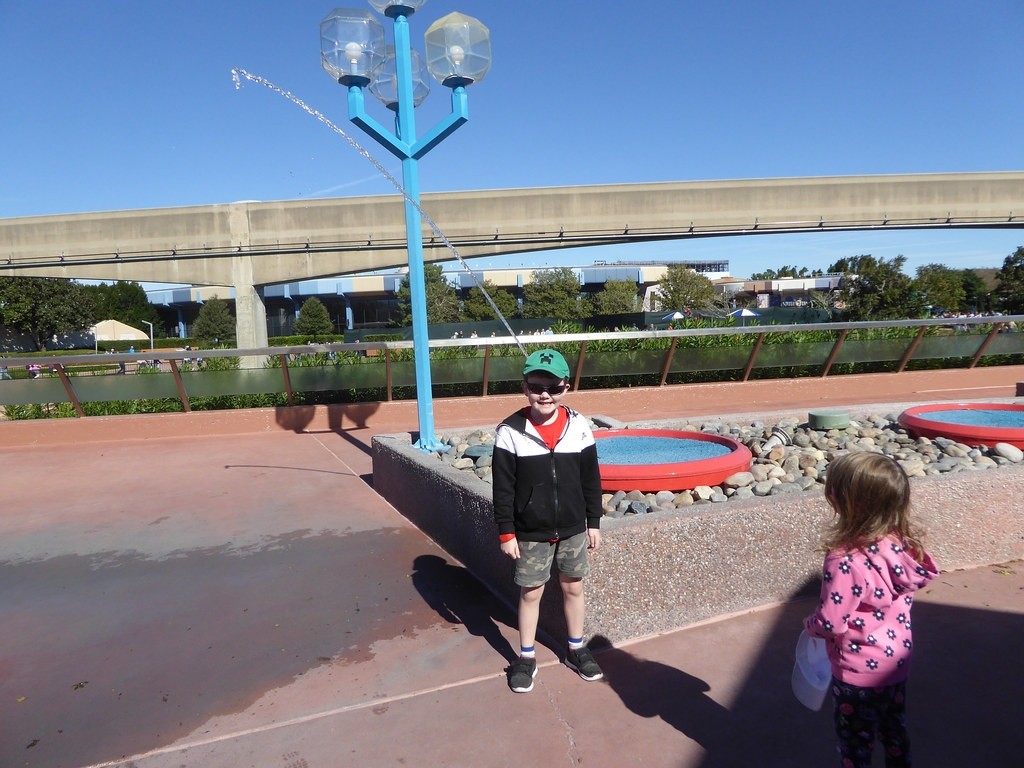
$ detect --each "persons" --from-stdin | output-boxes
[804,449,940,768]
[0,345,205,380]
[491,350,604,694]
[930,310,1018,334]
[450,325,557,358]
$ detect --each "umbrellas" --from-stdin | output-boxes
[726,308,763,327]
[662,312,684,326]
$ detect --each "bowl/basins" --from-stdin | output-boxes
[808,408,852,430]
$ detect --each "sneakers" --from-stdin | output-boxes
[510,657,538,693]
[564,647,603,680]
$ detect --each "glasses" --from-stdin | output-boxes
[525,381,566,395]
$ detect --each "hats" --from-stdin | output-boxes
[522,349,570,380]
[790,628,832,712]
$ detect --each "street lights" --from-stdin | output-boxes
[316,0,494,455]
